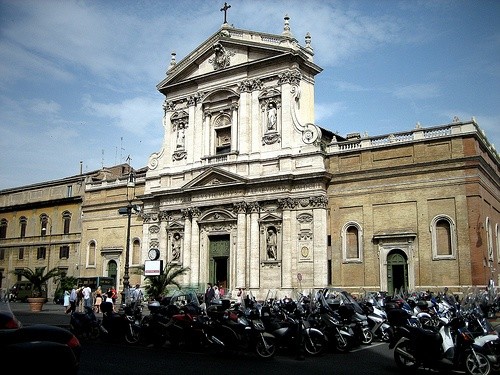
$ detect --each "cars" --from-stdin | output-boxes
[0,309,80,375]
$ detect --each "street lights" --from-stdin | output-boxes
[119,180,136,308]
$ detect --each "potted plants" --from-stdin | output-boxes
[8,264,70,312]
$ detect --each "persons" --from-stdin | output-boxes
[105,287,116,312]
[111,286,117,304]
[133,284,143,302]
[79,286,85,312]
[82,284,91,307]
[93,286,103,313]
[66,285,77,315]
[267,104,277,129]
[489,276,494,288]
[205,283,223,307]
[267,230,277,259]
[173,236,180,258]
[177,124,185,147]
[237,288,247,304]
[64,287,70,313]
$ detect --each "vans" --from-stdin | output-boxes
[10,281,48,303]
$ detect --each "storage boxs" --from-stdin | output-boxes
[384,302,399,313]
[207,305,225,319]
[326,298,340,311]
[387,307,408,326]
[100,302,114,313]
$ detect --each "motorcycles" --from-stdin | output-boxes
[67,284,500,375]
